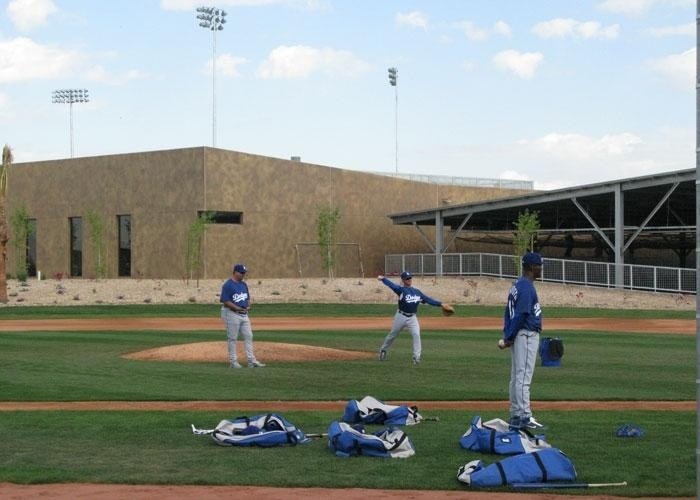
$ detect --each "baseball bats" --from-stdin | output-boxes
[514,483,629,489]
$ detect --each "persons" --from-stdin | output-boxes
[498,253,543,428]
[220,265,267,369]
[377,271,455,363]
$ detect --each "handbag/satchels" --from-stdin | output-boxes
[211,413,309,447]
[457,416,577,487]
[327,395,423,458]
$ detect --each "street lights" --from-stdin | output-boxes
[196,12,226,146]
[51,89,89,157]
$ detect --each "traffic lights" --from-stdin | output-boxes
[387,66,398,87]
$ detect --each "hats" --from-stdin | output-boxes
[401,272,412,279]
[522,253,542,265]
[234,265,247,273]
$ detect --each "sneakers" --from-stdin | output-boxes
[230,361,266,368]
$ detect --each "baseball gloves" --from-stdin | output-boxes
[443,303,455,315]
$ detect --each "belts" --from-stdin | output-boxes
[399,311,412,317]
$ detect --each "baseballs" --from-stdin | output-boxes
[498,339,504,346]
